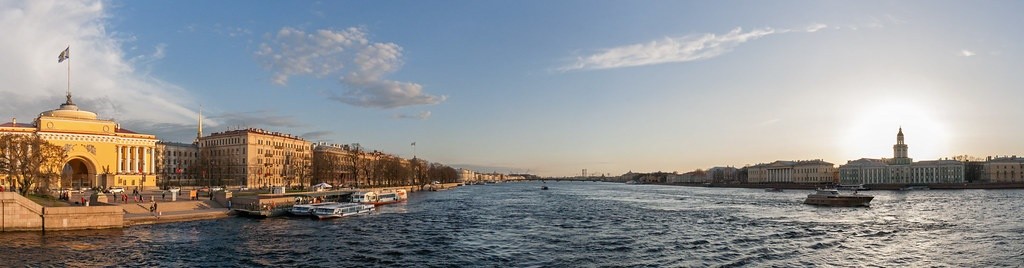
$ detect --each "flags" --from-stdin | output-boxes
[58,47,69,63]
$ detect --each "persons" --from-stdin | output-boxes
[122,194,124,201]
[162,191,165,199]
[59,192,69,200]
[154,202,158,213]
[113,192,117,202]
[125,193,128,204]
[134,195,138,202]
[196,192,198,200]
[81,195,85,206]
[209,192,213,200]
[139,195,143,202]
[227,200,231,210]
[150,205,154,213]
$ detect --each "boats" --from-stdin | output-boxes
[804,187,875,207]
[291,186,407,219]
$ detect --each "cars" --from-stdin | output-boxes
[109,186,125,194]
